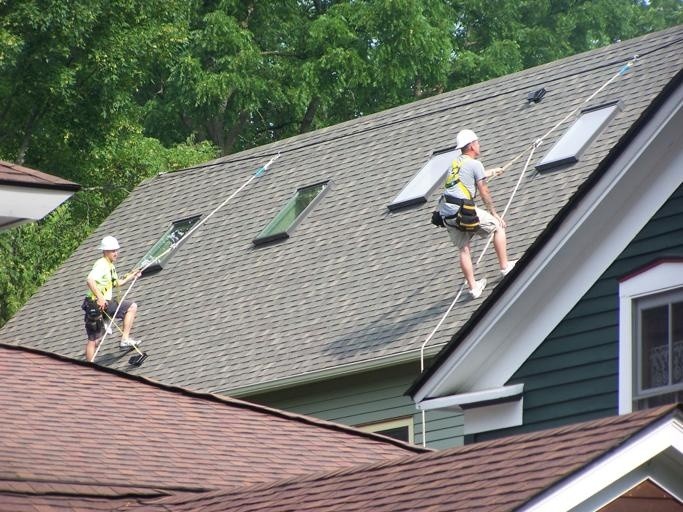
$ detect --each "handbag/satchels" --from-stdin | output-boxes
[455,199,480,231]
[85,311,104,333]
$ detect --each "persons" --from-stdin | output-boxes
[436,128,518,300]
[82,233,142,362]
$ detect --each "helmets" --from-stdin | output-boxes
[98,236,120,250]
[454,129,479,150]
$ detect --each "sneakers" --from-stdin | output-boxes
[120,338,142,348]
[498,260,518,277]
[469,277,487,300]
